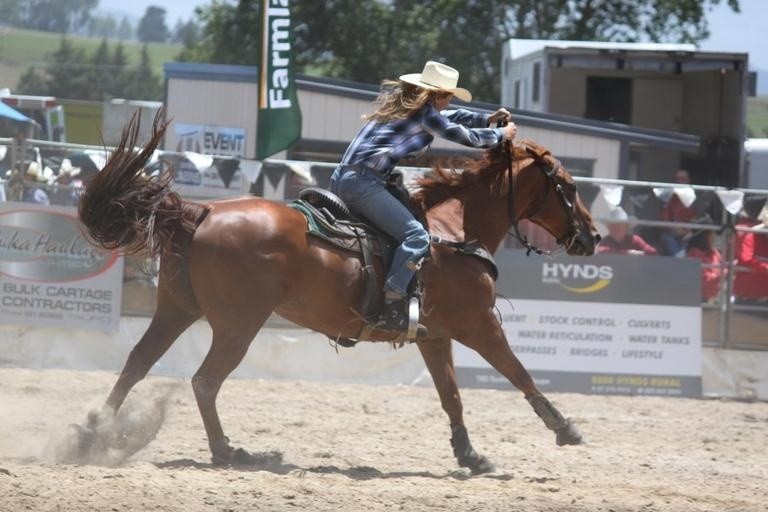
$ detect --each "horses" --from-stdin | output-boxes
[79,105,603,477]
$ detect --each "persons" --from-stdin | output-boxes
[659,169,694,223]
[591,207,656,254]
[324,55,516,339]
[650,215,695,259]
[682,212,728,304]
[7,158,51,206]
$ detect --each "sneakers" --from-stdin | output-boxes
[371,304,429,337]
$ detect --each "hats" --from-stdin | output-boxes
[5,160,46,182]
[44,157,82,179]
[397,58,474,104]
[683,211,724,241]
[598,205,639,227]
[674,169,690,178]
